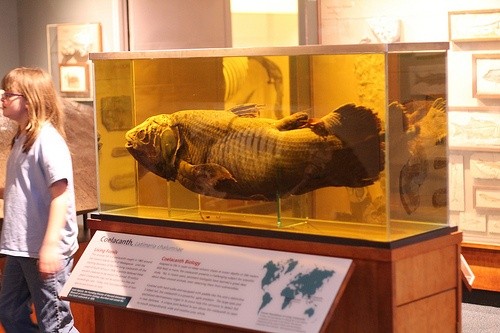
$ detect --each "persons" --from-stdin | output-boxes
[0,67,79,333]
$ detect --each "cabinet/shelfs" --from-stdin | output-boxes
[88,216,464,333]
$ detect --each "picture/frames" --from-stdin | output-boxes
[472,54,500,100]
[448,106,500,153]
[472,185,500,211]
[449,9,500,42]
[58,63,90,94]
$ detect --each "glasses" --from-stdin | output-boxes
[3,93,24,98]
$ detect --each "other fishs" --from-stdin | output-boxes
[125,104,385,201]
[450,116,500,139]
[387,92,449,216]
[414,72,446,85]
[482,68,500,84]
